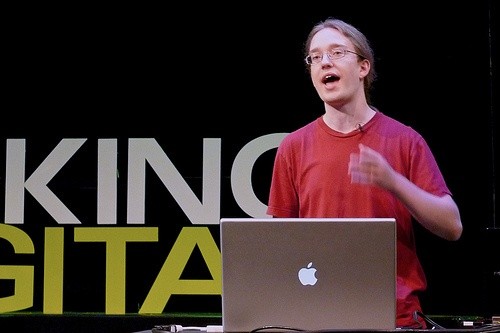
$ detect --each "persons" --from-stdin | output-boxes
[265,19,463,330]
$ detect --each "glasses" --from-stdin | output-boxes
[305,49,367,64]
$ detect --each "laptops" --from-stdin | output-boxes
[220,218,396,333]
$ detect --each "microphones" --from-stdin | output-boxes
[358,124,362,132]
